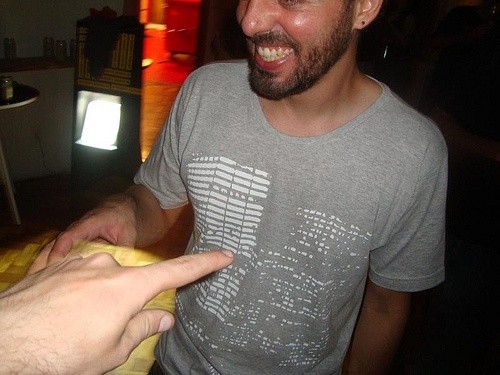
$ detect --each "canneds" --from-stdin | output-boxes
[0,76,17,102]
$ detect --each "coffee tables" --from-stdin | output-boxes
[0,82,45,226]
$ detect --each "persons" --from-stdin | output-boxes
[24,0,449,375]
[0,248,236,375]
